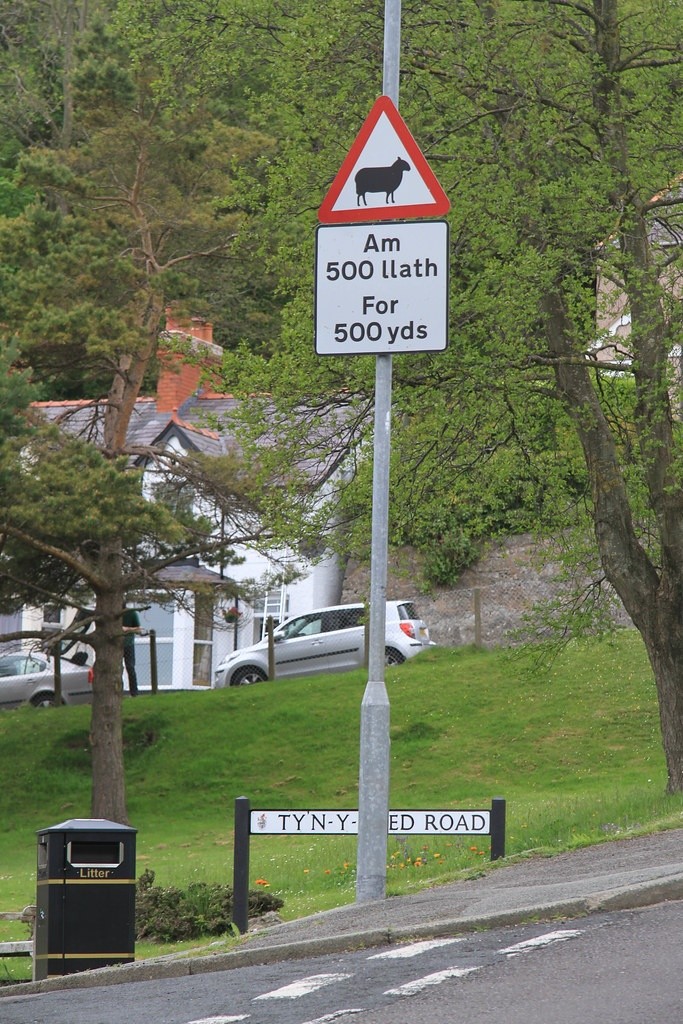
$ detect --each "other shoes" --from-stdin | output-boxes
[132,693,139,696]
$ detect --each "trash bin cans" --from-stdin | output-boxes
[30,819,140,980]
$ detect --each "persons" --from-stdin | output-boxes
[122,608,141,696]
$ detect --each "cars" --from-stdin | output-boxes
[214,600,436,690]
[0,652,94,707]
